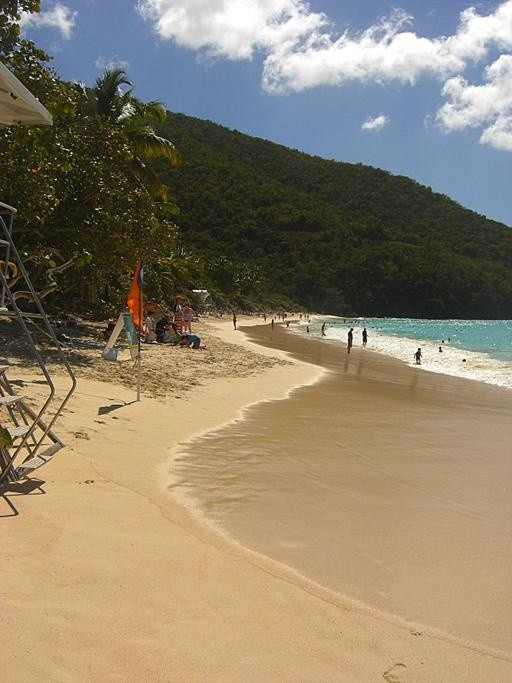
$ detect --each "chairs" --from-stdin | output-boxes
[145,318,182,345]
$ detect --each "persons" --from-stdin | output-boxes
[262,310,383,347]
[347,328,354,354]
[145,295,207,349]
[232,312,236,330]
[414,348,423,365]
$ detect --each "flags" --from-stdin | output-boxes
[125,264,145,330]
